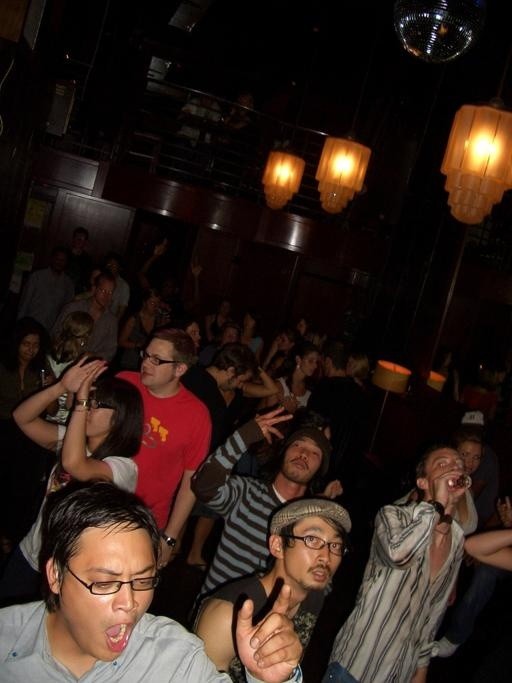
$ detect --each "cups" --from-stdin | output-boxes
[39,368,49,390]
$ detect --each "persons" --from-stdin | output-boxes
[218,91,256,144]
[178,85,223,146]
[1,225,511,682]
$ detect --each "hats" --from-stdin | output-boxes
[280,428,332,477]
[270,499,352,535]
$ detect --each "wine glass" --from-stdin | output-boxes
[445,474,471,495]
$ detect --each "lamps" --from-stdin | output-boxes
[261,78,308,211]
[393,0,478,63]
[439,48,512,226]
[314,30,383,218]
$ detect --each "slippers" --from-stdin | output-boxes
[186,561,208,572]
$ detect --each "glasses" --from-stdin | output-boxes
[66,564,159,595]
[281,534,349,557]
[140,350,183,365]
[98,287,114,295]
[303,358,319,363]
[88,398,114,410]
